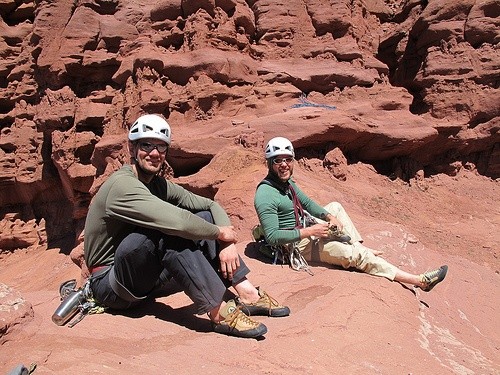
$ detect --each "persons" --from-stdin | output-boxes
[258,137,448,293]
[84,115,290,338]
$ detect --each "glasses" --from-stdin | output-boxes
[273,160,291,163]
[138,143,167,152]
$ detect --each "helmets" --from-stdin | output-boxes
[129,114,172,143]
[265,138,295,158]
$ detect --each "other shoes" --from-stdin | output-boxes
[236,286,290,317]
[320,225,352,243]
[419,264,447,292]
[209,299,267,338]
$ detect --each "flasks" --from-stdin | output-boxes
[52,287,86,325]
